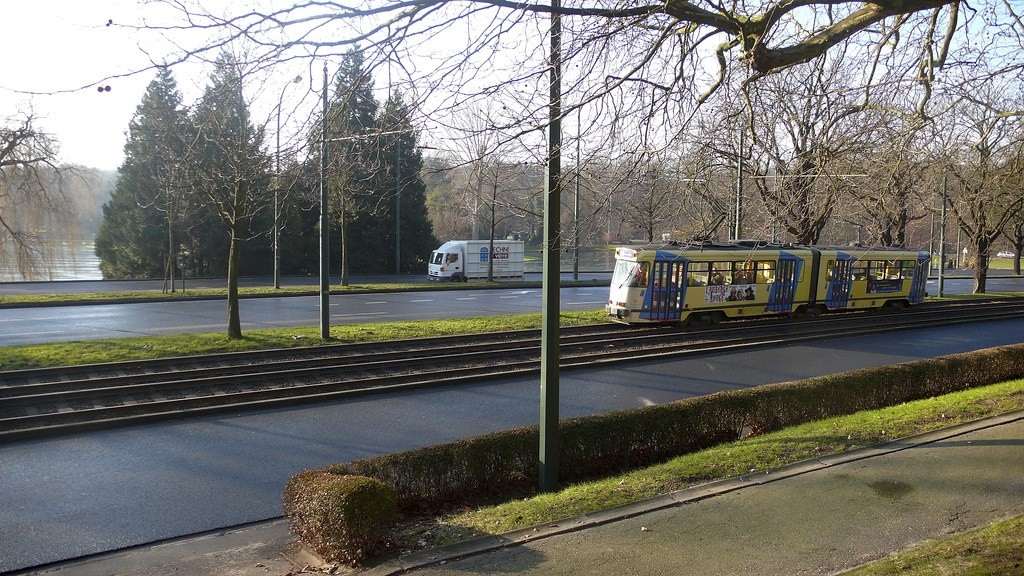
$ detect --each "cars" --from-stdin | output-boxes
[997,250,1015,259]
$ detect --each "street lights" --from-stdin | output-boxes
[272,75,303,286]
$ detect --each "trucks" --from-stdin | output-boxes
[427,240,525,283]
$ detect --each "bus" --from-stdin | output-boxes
[606,238,932,331]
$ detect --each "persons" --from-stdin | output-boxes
[964,258,969,268]
[725,286,755,301]
[948,258,957,269]
